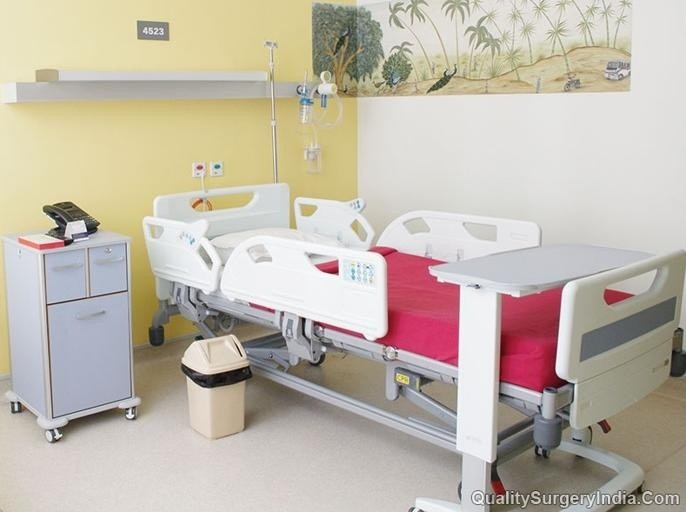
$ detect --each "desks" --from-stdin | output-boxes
[411,242,658,511]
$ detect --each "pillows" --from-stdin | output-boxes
[201,228,305,266]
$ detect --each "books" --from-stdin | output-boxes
[18,233,65,249]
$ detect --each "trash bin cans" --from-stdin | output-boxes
[181,335,253,439]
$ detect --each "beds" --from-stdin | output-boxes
[142,182,685,511]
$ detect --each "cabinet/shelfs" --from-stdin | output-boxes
[3,228,142,444]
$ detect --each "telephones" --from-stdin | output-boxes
[43,202,100,239]
